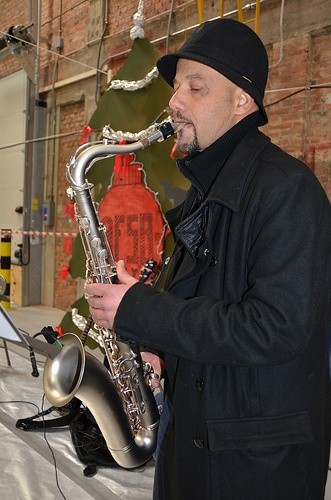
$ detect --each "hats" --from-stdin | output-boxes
[156,18,268,128]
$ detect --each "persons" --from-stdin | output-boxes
[85,17,331,500]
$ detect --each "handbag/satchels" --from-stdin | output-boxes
[14,397,120,477]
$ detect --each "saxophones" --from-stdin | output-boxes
[41,115,184,470]
